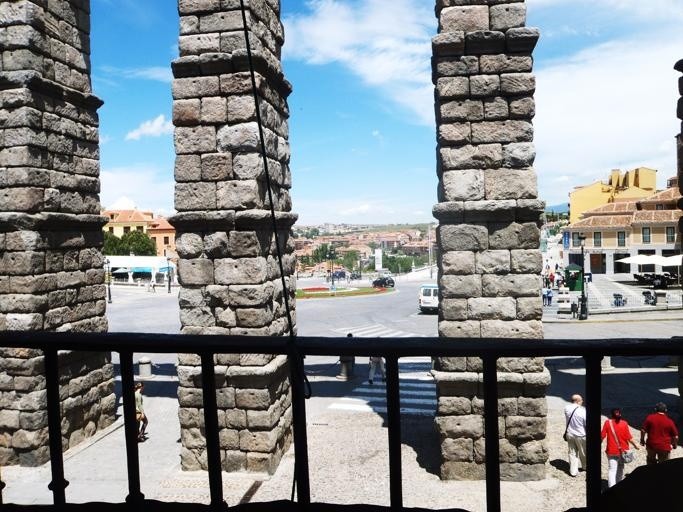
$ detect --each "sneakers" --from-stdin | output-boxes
[138,434,146,442]
[368,379,373,385]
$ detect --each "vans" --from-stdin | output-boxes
[418,284,439,313]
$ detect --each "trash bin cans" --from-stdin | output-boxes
[144,283,150,292]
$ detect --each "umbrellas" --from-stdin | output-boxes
[615,253,683,287]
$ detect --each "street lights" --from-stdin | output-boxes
[325,251,331,277]
[105,258,112,303]
[329,246,334,286]
[165,256,172,294]
[577,232,587,321]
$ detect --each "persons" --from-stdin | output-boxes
[340,333,356,379]
[542,255,579,319]
[640,402,680,465]
[135,383,150,441]
[601,407,642,485]
[369,356,388,384]
[151,280,156,293]
[564,394,587,478]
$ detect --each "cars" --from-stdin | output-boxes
[349,270,361,280]
[331,270,344,279]
[370,276,395,288]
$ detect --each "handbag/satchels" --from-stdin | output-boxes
[563,405,579,441]
[135,413,142,421]
[609,418,634,463]
